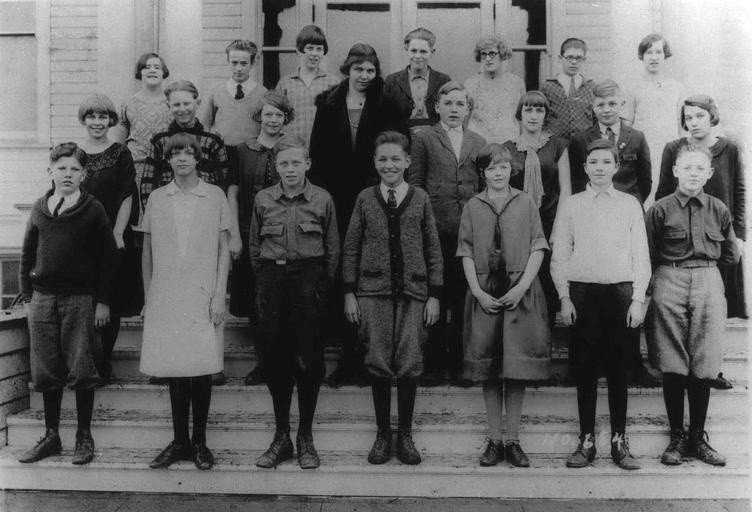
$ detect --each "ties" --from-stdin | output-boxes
[569,79,576,97]
[607,128,615,145]
[387,189,397,207]
[235,85,244,99]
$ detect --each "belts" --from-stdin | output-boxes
[668,259,717,268]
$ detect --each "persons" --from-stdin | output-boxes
[136,132,232,472]
[16,142,120,467]
[454,143,552,466]
[247,137,340,470]
[548,138,652,471]
[343,130,445,464]
[20,22,749,391]
[644,143,741,468]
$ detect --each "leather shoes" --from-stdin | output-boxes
[611,431,642,469]
[397,428,421,465]
[368,427,393,464]
[479,437,504,466]
[245,366,264,385]
[505,442,530,467]
[688,426,726,466]
[192,438,214,470]
[211,373,226,386]
[711,373,733,389]
[561,373,578,387]
[636,370,662,388]
[567,432,597,467]
[661,427,688,465]
[72,431,94,465]
[150,439,191,469]
[256,432,294,468]
[19,427,61,462]
[297,433,320,469]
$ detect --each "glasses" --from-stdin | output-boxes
[481,51,499,60]
[563,55,583,62]
